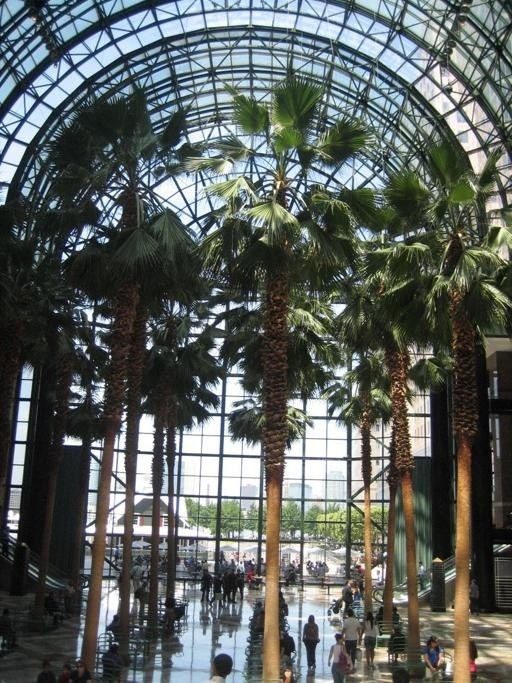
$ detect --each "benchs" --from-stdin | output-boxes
[345,594,452,682]
[0,586,88,657]
[243,596,301,682]
[61,589,190,682]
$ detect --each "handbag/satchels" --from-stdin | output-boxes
[338,645,356,674]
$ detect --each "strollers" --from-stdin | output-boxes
[328,597,344,616]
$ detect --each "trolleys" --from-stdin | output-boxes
[249,576,264,591]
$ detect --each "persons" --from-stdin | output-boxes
[375,606,405,662]
[101,533,244,604]
[328,633,353,682]
[468,638,477,680]
[280,632,295,658]
[137,582,147,619]
[73,659,92,682]
[302,615,318,669]
[469,578,479,617]
[101,614,131,683]
[44,587,75,627]
[1,607,19,646]
[337,563,377,670]
[280,667,294,682]
[417,561,426,593]
[58,663,72,683]
[421,634,444,683]
[239,555,329,586]
[392,667,410,682]
[211,653,233,682]
[36,658,57,682]
[160,596,181,669]
